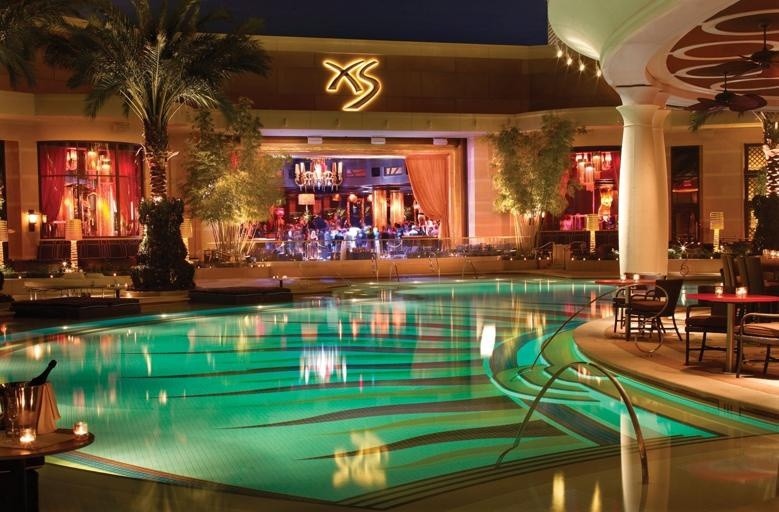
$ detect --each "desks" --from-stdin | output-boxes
[595,277,656,336]
[0,429,95,510]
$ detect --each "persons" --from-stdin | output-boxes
[266,215,440,260]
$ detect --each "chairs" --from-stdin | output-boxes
[612,252,779,378]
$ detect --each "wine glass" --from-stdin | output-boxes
[6,410,19,435]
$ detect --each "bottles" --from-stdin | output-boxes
[26,360,57,385]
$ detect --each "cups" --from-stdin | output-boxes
[18,426,36,443]
[619,274,640,282]
[715,287,723,297]
[762,249,779,258]
[735,287,747,298]
[73,422,89,441]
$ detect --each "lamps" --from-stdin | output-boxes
[178,216,193,264]
[63,217,84,270]
[0,220,10,268]
[587,214,598,253]
[710,211,723,250]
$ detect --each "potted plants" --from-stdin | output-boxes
[48,4,272,290]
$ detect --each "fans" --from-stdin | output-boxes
[685,22,779,112]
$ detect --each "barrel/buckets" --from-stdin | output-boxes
[0,380,44,436]
